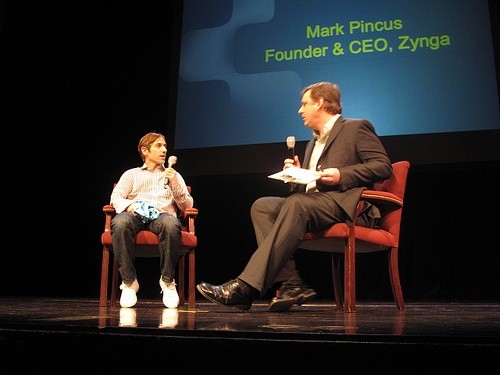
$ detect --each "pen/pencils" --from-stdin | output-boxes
[318,165,324,171]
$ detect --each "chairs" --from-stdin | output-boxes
[277,160,410,311]
[99,183,198,307]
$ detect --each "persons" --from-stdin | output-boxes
[196,81,393,312]
[108,132,193,308]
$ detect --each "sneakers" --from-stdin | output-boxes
[119,277,140,308]
[161,308,179,328]
[159,275,180,308]
[120,308,137,327]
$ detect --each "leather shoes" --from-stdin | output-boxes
[197,280,254,309]
[267,285,317,311]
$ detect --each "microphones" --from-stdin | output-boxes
[164,155,177,189]
[287,136,296,165]
